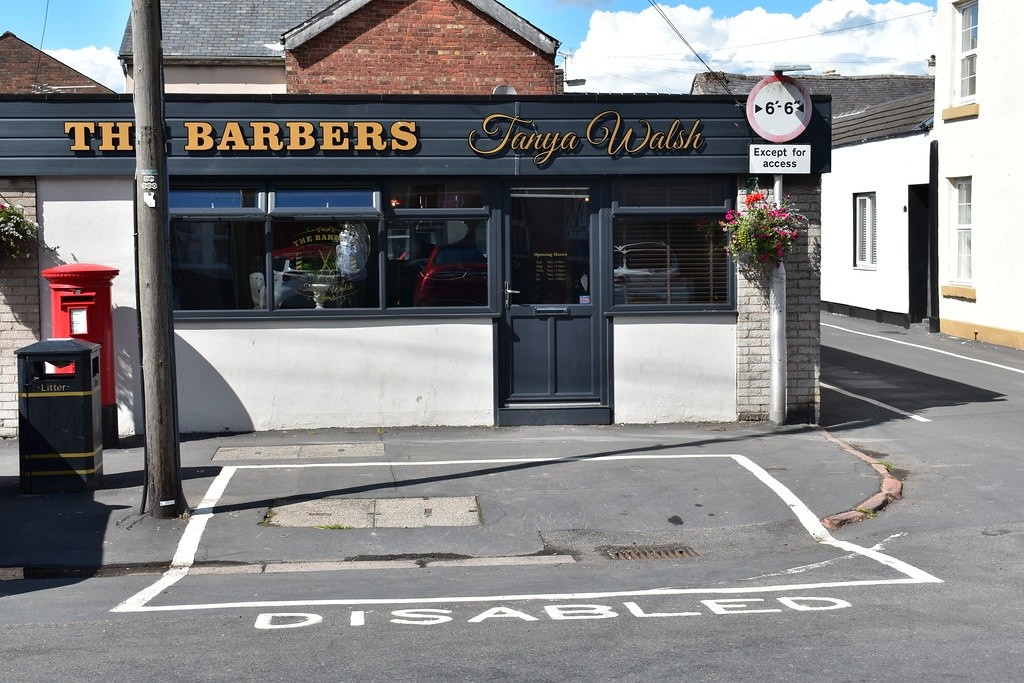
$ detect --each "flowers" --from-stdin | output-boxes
[717,181,812,279]
[0,192,61,262]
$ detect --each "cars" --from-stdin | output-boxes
[614,243,693,306]
[273,270,346,308]
[411,241,487,306]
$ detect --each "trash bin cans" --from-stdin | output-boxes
[14,338,105,493]
